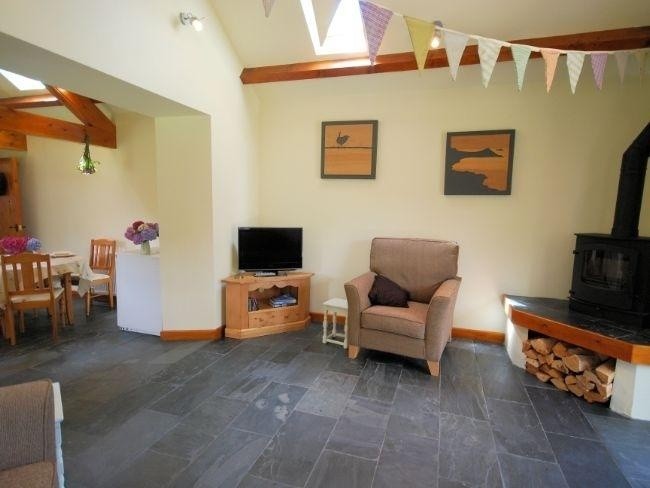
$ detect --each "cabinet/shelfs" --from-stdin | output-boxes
[221,270,314,339]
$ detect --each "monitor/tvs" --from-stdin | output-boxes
[236,226,303,277]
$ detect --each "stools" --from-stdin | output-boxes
[320,297,350,351]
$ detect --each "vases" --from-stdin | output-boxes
[140,241,151,256]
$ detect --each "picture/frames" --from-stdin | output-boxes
[320,119,379,181]
[444,129,517,195]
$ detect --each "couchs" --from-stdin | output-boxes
[342,237,463,377]
[0,381,69,488]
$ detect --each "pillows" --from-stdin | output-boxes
[368,274,411,309]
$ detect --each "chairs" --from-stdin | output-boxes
[60,238,117,316]
[4,252,67,346]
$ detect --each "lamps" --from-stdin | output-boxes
[180,12,204,30]
[75,134,101,177]
[429,19,444,48]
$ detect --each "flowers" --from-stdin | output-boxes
[124,220,159,245]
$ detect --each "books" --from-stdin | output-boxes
[269,294,297,307]
